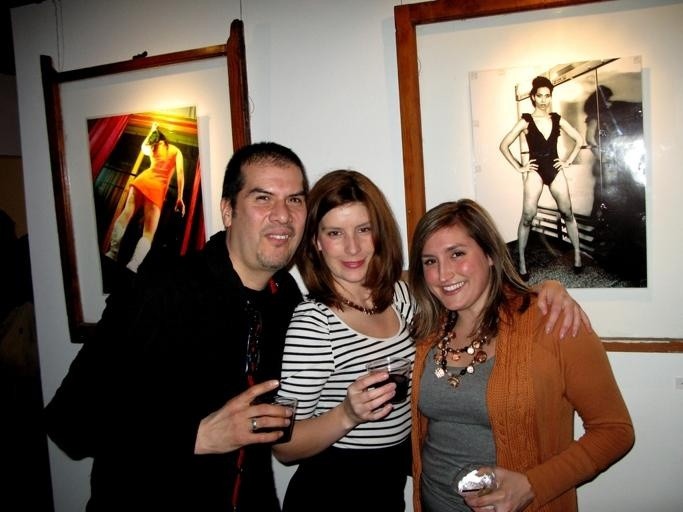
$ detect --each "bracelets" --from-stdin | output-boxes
[566,160,573,167]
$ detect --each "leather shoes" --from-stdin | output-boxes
[573,264,583,275]
[519,270,530,282]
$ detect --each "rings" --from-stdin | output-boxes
[491,503,500,512]
[251,417,259,434]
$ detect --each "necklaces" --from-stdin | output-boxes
[332,289,379,315]
[432,308,492,387]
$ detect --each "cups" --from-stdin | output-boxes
[366,358,411,405]
[451,462,498,499]
[259,397,297,445]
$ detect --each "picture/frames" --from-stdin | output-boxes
[389,0,681,355]
[37,18,257,345]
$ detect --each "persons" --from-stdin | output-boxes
[271,169,594,511]
[500,76,583,282]
[45,144,307,512]
[410,196,634,511]
[584,85,614,220]
[107,121,187,275]
